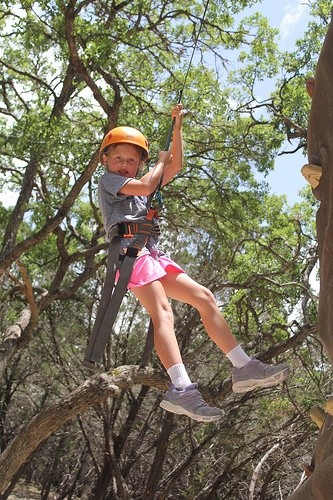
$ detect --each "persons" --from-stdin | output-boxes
[98,103,290,423]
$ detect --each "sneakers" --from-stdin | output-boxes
[160,382,225,422]
[231,358,289,393]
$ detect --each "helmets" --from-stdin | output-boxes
[99,126,149,161]
